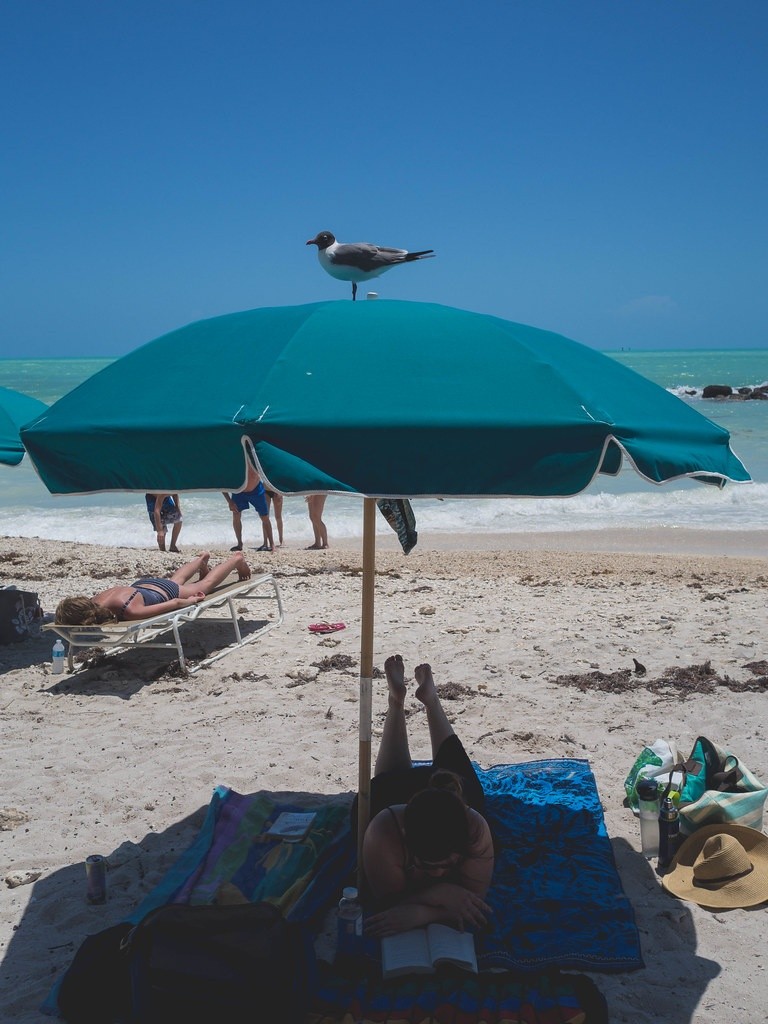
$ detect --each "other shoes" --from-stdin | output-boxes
[230,546,242,551]
[257,546,272,551]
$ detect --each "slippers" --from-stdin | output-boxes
[308,620,344,632]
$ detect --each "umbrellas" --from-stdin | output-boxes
[0,385,52,465]
[18,299,753,902]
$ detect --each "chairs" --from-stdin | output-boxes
[40,572,284,676]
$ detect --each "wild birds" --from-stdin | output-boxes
[306,231,436,301]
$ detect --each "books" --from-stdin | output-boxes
[381,923,478,981]
[267,812,316,839]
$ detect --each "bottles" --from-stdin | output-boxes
[636,781,660,858]
[52,639,65,674]
[659,798,680,867]
[338,887,363,956]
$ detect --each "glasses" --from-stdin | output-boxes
[409,846,463,870]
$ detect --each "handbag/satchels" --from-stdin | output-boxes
[623,735,768,836]
[0,590,41,643]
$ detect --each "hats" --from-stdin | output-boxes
[663,823,768,908]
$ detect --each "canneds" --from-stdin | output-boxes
[86,854,106,905]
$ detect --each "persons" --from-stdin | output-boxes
[262,480,284,549]
[350,654,495,936]
[145,494,183,552]
[56,553,250,624]
[304,494,329,550]
[222,459,276,552]
